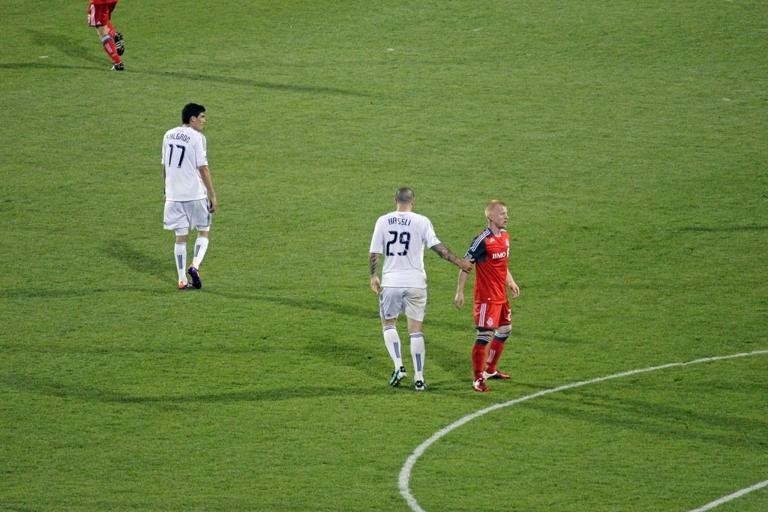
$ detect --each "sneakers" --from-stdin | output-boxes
[483,370,511,380]
[178,280,193,289]
[110,62,124,70]
[389,366,408,387]
[414,379,427,391]
[113,31,125,57]
[188,264,202,289]
[472,378,489,393]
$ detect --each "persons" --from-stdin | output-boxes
[161,102,216,289]
[452,201,522,391]
[88,1,126,70]
[371,187,477,391]
